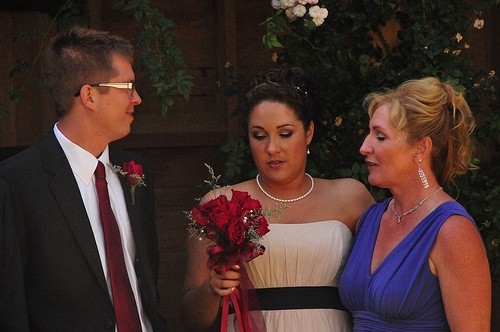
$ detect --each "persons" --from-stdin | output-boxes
[0,26,167,332]
[181,68,376,332]
[338,77,500,332]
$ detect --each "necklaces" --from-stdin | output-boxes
[391,188,443,223]
[256,173,314,201]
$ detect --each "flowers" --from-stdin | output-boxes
[180,162,291,332]
[107,161,148,208]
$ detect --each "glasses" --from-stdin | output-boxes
[74,81,136,100]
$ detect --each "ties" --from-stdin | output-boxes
[92,160,143,332]
